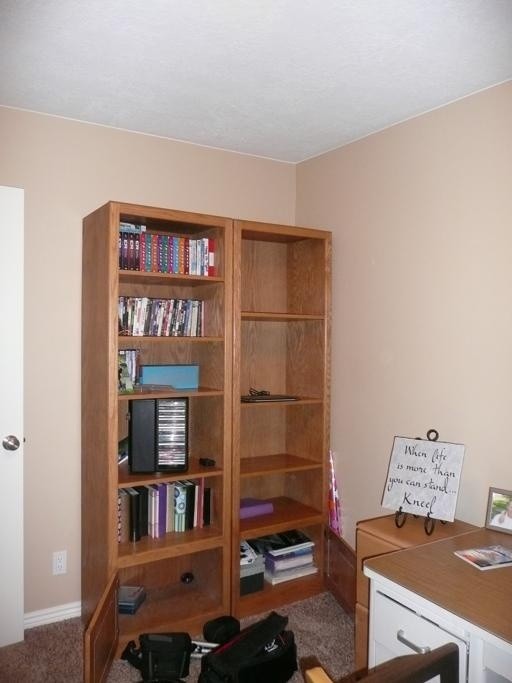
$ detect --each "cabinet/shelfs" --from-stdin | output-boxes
[82,201,359,683]
[354,514,481,672]
[363,530,512,682]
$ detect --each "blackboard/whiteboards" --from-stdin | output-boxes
[381,436,465,522]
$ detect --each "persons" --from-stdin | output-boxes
[489,500,512,530]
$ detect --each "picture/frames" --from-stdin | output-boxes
[485,486,512,535]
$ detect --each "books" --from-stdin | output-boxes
[453,544,512,571]
[240,498,274,520]
[240,529,318,596]
[119,223,215,277]
[119,585,147,614]
[118,349,176,395]
[118,477,210,545]
[240,396,299,402]
[118,296,205,337]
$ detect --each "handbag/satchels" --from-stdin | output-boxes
[197,611,298,683]
[120,632,197,683]
